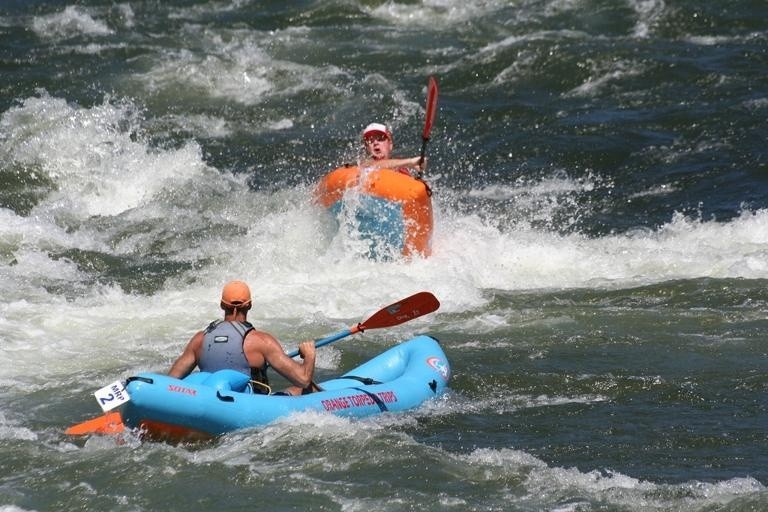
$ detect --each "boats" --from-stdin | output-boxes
[311,165,432,261]
[119,334,450,439]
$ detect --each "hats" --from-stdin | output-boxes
[221,281,251,318]
[362,122,392,139]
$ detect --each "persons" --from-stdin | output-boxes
[360,122,434,190]
[168,281,322,398]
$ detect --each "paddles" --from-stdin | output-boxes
[418,76,437,178]
[66,292,440,436]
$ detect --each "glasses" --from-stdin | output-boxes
[365,134,389,143]
